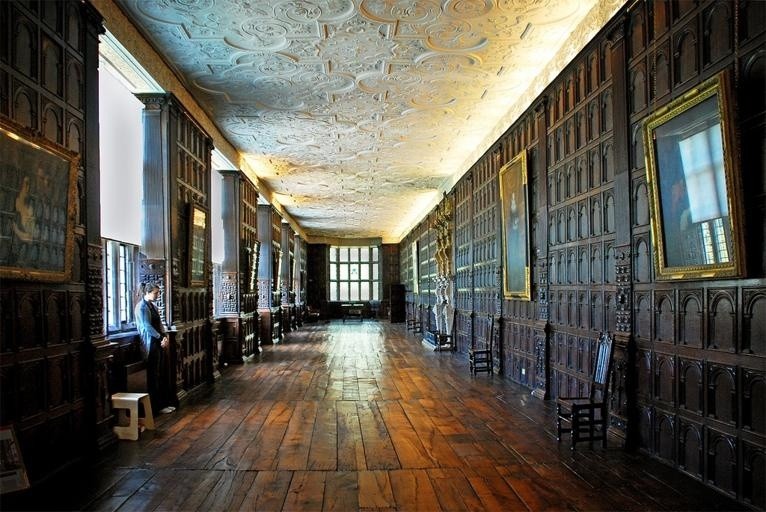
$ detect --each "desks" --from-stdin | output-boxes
[342,304,364,323]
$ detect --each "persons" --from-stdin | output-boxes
[11,169,36,268]
[508,189,522,292]
[133,282,176,413]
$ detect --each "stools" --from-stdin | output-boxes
[110,392,155,441]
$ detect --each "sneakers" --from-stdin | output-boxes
[168,406,176,411]
[159,407,172,413]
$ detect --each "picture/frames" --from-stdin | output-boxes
[0,116,81,282]
[274,250,283,292]
[640,69,742,284]
[184,200,209,288]
[0,424,31,494]
[247,239,262,292]
[497,149,533,303]
[412,240,420,296]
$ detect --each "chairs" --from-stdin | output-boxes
[406,303,616,452]
